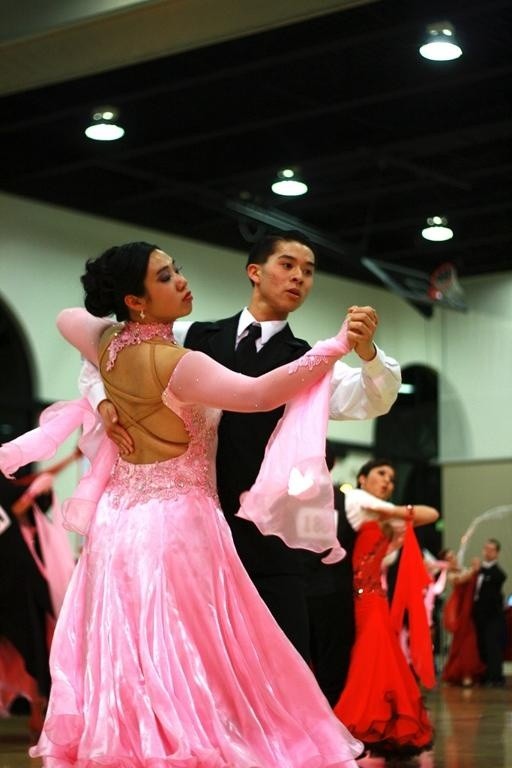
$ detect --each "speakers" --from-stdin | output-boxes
[374,361,440,458]
[390,461,440,556]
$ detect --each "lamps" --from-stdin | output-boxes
[269,164,308,197]
[84,104,126,142]
[418,19,463,60]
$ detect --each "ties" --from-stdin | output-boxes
[237,326,262,356]
